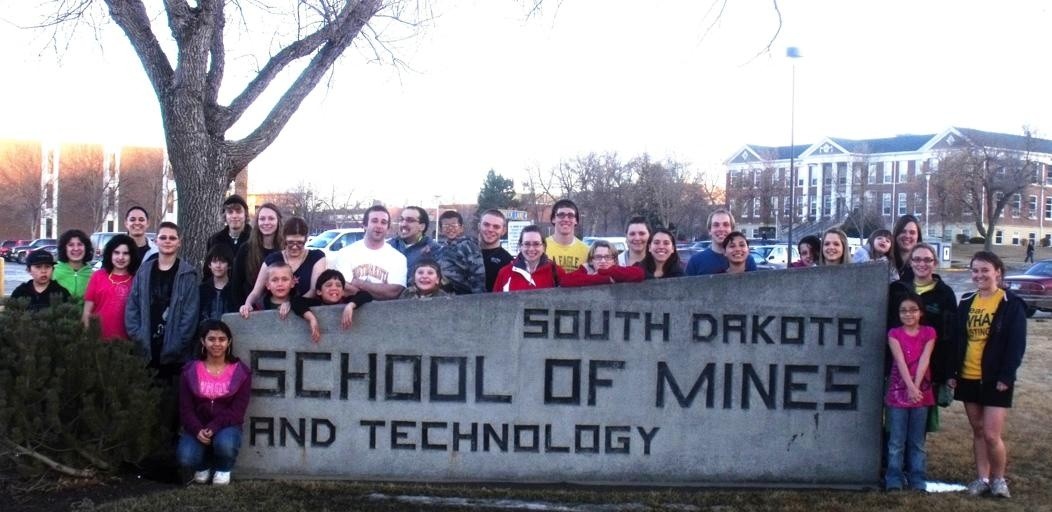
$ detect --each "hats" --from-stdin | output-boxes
[550,199,579,226]
[26,250,58,265]
[223,195,248,215]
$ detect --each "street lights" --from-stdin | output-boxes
[580,211,585,238]
[785,37,805,268]
[923,170,934,236]
[433,193,443,245]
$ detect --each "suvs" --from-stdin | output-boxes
[85,231,156,262]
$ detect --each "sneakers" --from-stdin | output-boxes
[991,479,1011,498]
[212,471,230,485]
[967,477,989,495]
[193,468,210,484]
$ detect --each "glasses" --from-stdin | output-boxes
[159,234,176,241]
[900,308,918,314]
[912,256,935,263]
[522,241,541,248]
[287,241,306,248]
[592,254,614,261]
[555,212,575,220]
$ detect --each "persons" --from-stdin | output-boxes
[683,207,756,276]
[80,234,141,340]
[946,251,1034,498]
[50,229,94,301]
[882,294,939,498]
[125,221,200,388]
[615,215,688,278]
[125,206,159,266]
[1024,241,1034,264]
[9,249,73,312]
[888,214,924,274]
[198,196,370,343]
[816,228,853,266]
[560,240,645,287]
[175,321,251,486]
[850,228,901,285]
[723,231,750,274]
[886,244,957,485]
[792,234,821,268]
[335,198,590,301]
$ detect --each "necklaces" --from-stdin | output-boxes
[109,271,131,285]
[212,361,225,374]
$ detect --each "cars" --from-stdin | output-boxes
[305,228,366,259]
[582,236,628,266]
[1003,259,1052,317]
[675,238,803,272]
[849,244,861,256]
[1,237,63,267]
